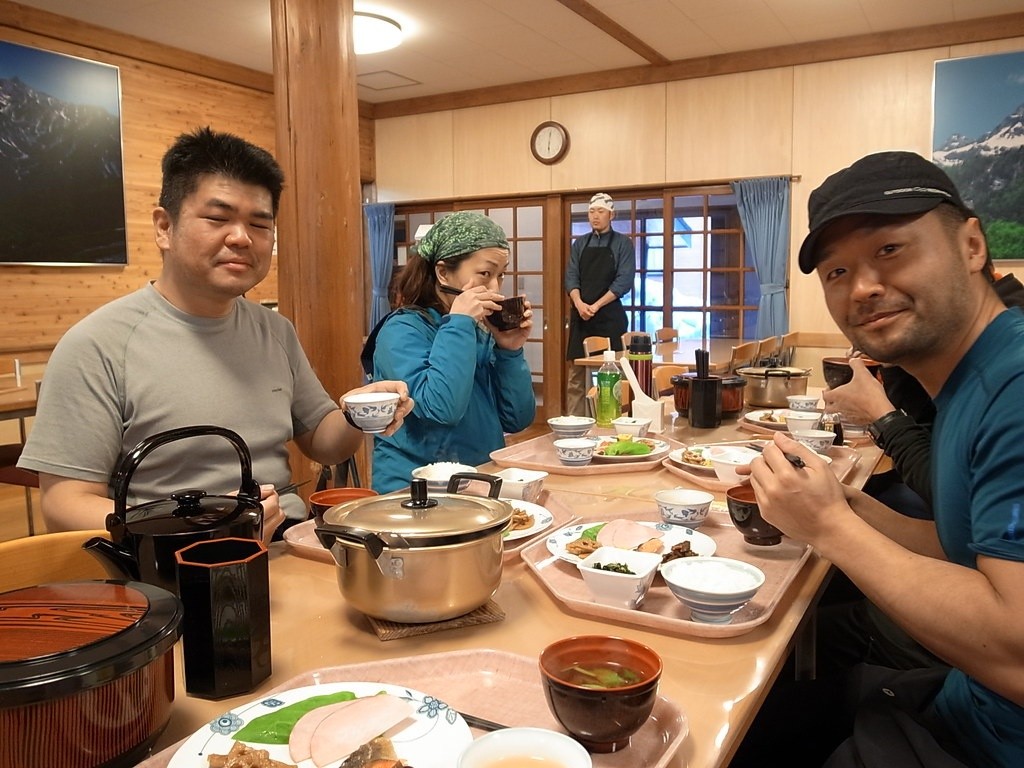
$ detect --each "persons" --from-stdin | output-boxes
[359,210,537,495]
[15,123,414,550]
[564,192,635,417]
[735,151,1024,768]
[783,260,1024,757]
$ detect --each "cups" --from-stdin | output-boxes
[174,538,273,700]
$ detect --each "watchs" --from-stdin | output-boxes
[866,408,908,443]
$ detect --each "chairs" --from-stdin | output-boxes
[0,358,128,590]
[582,328,798,418]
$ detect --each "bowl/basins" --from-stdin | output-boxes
[576,547,663,612]
[726,485,786,546]
[491,467,550,504]
[656,488,714,528]
[344,392,401,434]
[457,727,593,768]
[485,297,525,331]
[548,357,883,484]
[658,556,766,625]
[412,465,476,493]
[539,635,662,754]
[309,487,379,526]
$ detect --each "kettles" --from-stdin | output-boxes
[81,424,264,604]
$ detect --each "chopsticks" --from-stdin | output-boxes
[696,348,710,380]
[457,712,507,732]
[744,443,806,468]
[440,284,463,296]
[277,479,311,496]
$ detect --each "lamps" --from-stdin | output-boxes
[352,10,404,54]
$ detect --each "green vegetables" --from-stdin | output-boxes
[605,441,650,455]
[581,522,608,540]
[230,690,386,744]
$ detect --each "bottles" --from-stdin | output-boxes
[628,335,652,418]
[597,351,621,428]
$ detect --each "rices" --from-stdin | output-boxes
[666,560,757,593]
[414,461,472,481]
[550,414,591,424]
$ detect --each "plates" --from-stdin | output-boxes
[499,498,555,543]
[546,520,715,570]
[166,681,474,768]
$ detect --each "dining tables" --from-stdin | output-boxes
[171,385,888,768]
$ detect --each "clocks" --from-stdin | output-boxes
[530,121,567,163]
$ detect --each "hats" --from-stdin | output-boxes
[798,151,963,274]
[588,194,616,212]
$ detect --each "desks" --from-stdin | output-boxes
[574,338,781,376]
[0,371,42,421]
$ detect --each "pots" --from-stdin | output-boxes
[671,372,746,417]
[314,472,514,623]
[0,580,185,768]
[735,358,811,409]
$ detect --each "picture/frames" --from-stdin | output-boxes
[0,39,129,267]
[929,48,1024,262]
